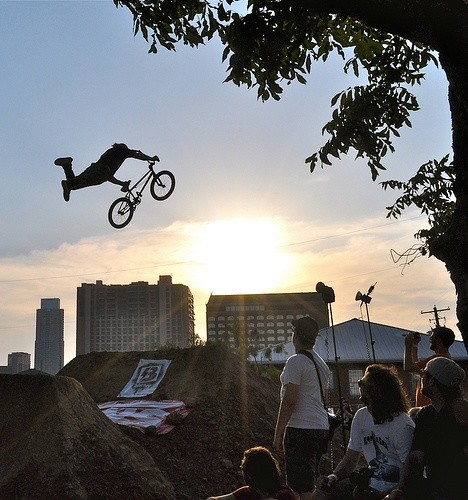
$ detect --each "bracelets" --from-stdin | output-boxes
[391,488,404,496]
[413,344,419,348]
[328,473,338,481]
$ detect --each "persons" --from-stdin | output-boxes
[273,316,331,500]
[53,143,161,202]
[310,327,468,500]
[206,446,297,500]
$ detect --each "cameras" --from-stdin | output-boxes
[413,334,419,340]
[327,412,341,442]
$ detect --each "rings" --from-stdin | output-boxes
[385,494,390,499]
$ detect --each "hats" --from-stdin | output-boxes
[424,357,465,390]
[431,327,455,346]
[291,316,318,335]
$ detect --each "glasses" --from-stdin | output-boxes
[292,327,296,332]
[358,379,368,391]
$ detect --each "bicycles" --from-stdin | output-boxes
[108,159,176,229]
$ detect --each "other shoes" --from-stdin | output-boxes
[55,157,73,165]
[62,180,71,201]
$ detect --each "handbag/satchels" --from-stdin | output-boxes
[328,415,336,439]
